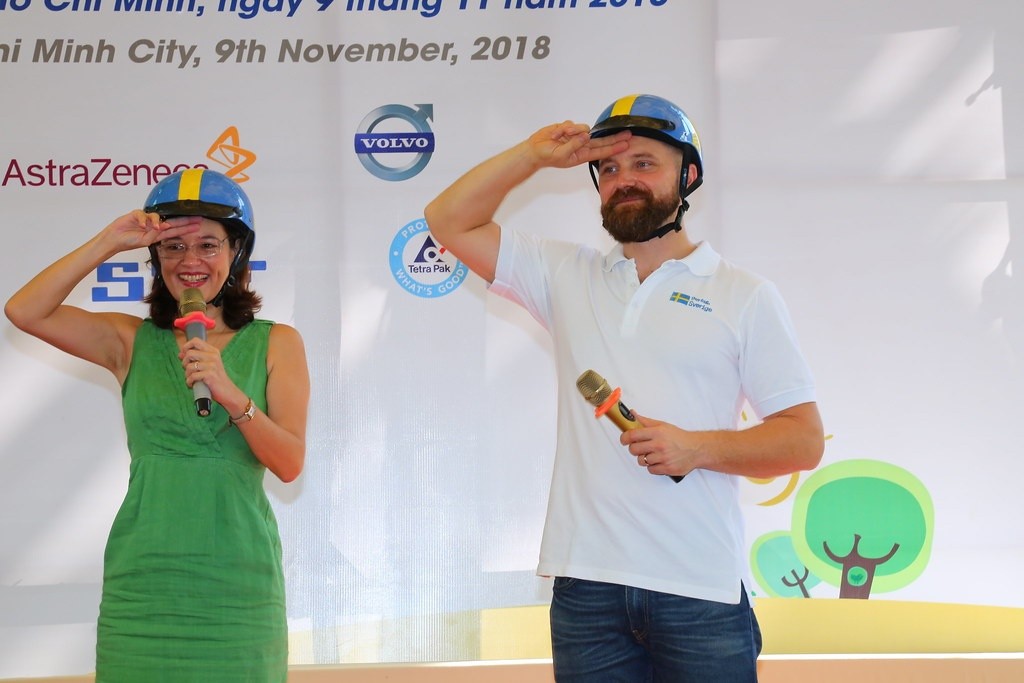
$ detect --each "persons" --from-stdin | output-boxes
[423,94,824,683]
[4,168,309,683]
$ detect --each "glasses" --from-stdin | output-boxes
[154,235,233,259]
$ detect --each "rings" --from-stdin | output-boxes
[644,455,649,465]
[195,362,199,371]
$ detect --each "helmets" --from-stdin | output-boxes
[143,168,255,261]
[585,92,702,199]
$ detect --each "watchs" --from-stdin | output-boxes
[228,398,256,427]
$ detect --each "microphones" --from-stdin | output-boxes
[576,370,685,483]
[174,287,215,417]
